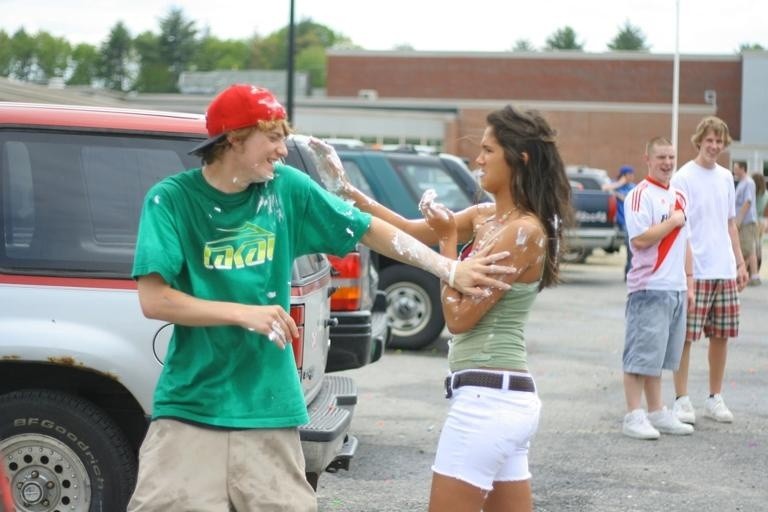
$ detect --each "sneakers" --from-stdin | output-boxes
[673,396,694,424]
[646,406,693,436]
[622,409,659,440]
[702,393,732,422]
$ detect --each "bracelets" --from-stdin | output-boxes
[736,260,746,268]
[450,259,460,287]
[685,273,695,277]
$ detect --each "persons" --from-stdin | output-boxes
[308,105,573,510]
[669,115,748,423]
[732,161,761,284]
[624,136,694,440]
[602,167,637,282]
[126,83,516,512]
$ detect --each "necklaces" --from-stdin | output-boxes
[468,203,520,259]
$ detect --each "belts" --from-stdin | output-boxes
[443,372,534,398]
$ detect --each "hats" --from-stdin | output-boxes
[619,166,632,175]
[186,84,286,157]
